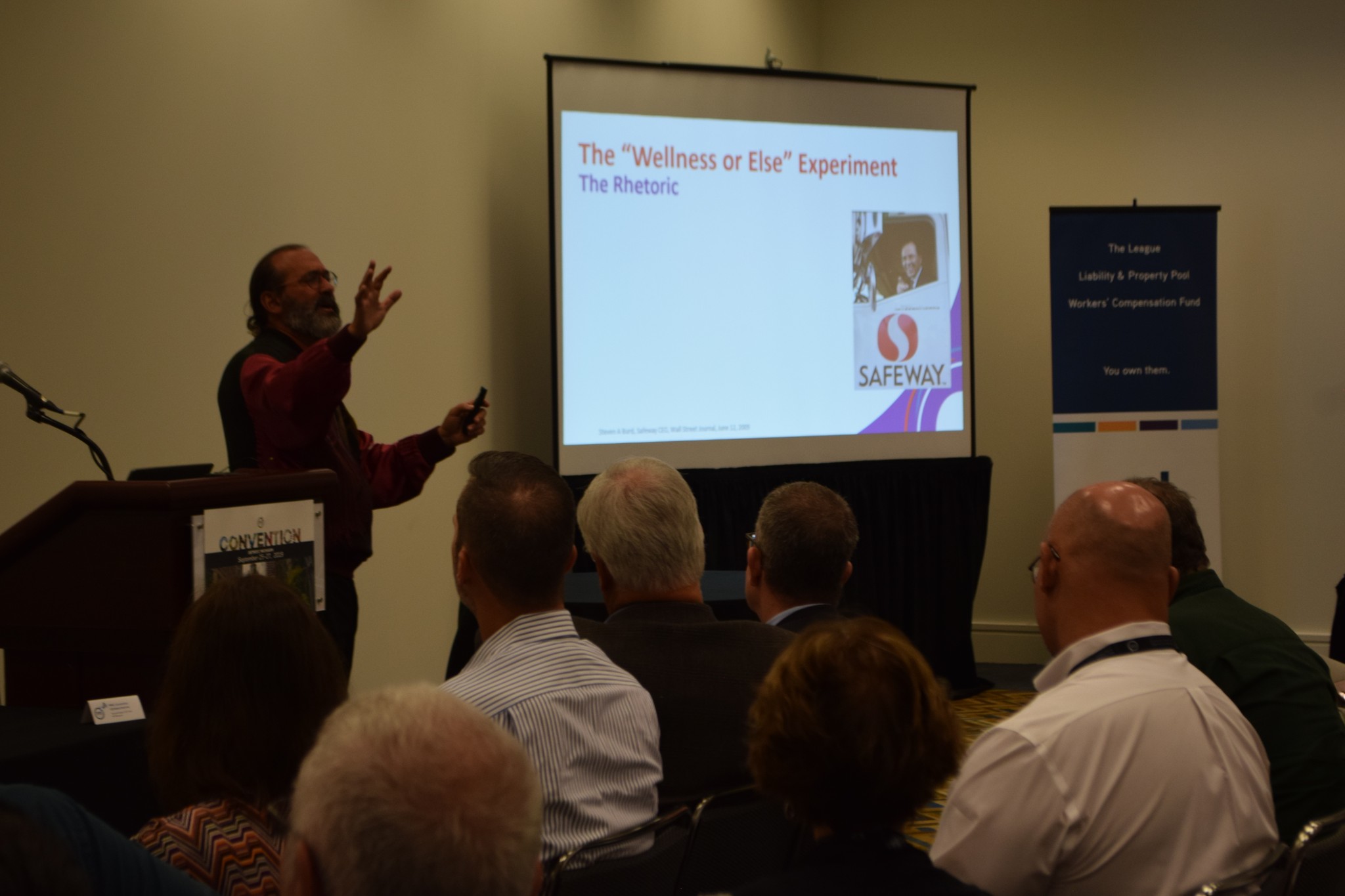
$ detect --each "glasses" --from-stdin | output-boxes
[277,267,339,291]
[1029,542,1063,582]
[745,531,771,557]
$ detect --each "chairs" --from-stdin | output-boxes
[533,785,792,896]
[1193,808,1345,896]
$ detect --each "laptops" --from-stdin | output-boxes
[129,462,215,480]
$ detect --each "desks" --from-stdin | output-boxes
[441,569,756,674]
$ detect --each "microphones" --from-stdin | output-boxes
[0,364,65,414]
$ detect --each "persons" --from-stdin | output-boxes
[216,243,490,697]
[896,240,937,294]
[929,476,1345,896]
[670,614,991,896]
[571,456,860,818]
[0,573,541,896]
[433,448,665,877]
